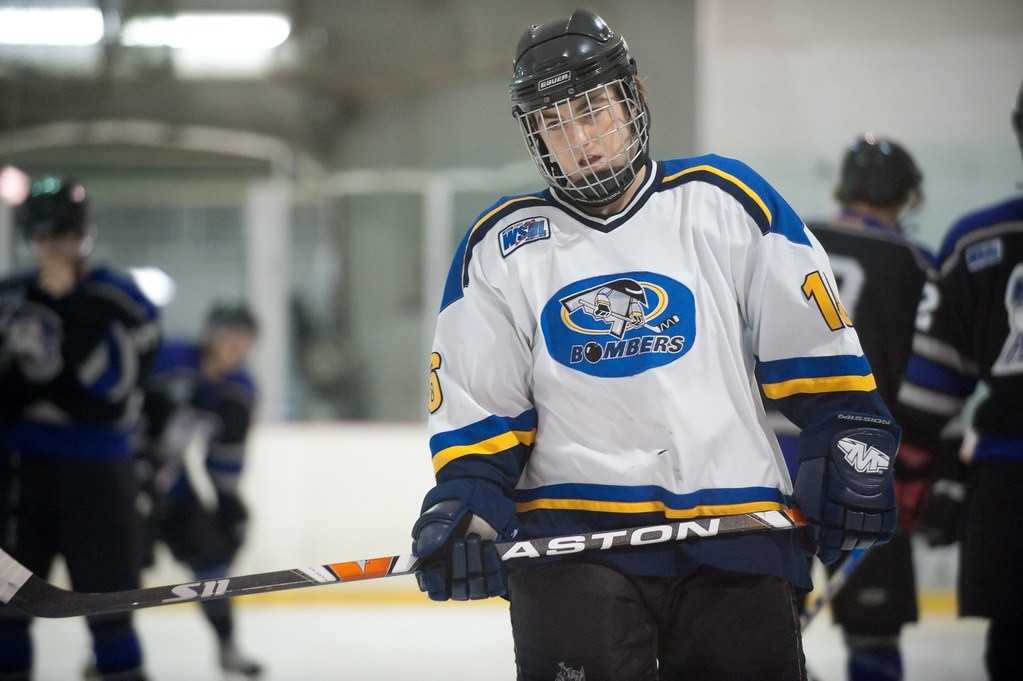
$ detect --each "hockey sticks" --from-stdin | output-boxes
[0,508,807,620]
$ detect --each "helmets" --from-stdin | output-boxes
[509,8,651,207]
[836,140,920,209]
[206,296,254,339]
[14,176,90,236]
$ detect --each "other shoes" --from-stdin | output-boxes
[220,653,259,676]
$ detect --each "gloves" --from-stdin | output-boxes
[793,412,902,564]
[412,500,522,601]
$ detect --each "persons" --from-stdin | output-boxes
[141,299,266,681]
[890,80,1023,681]
[411,9,903,681]
[0,177,172,681]
[757,135,969,681]
